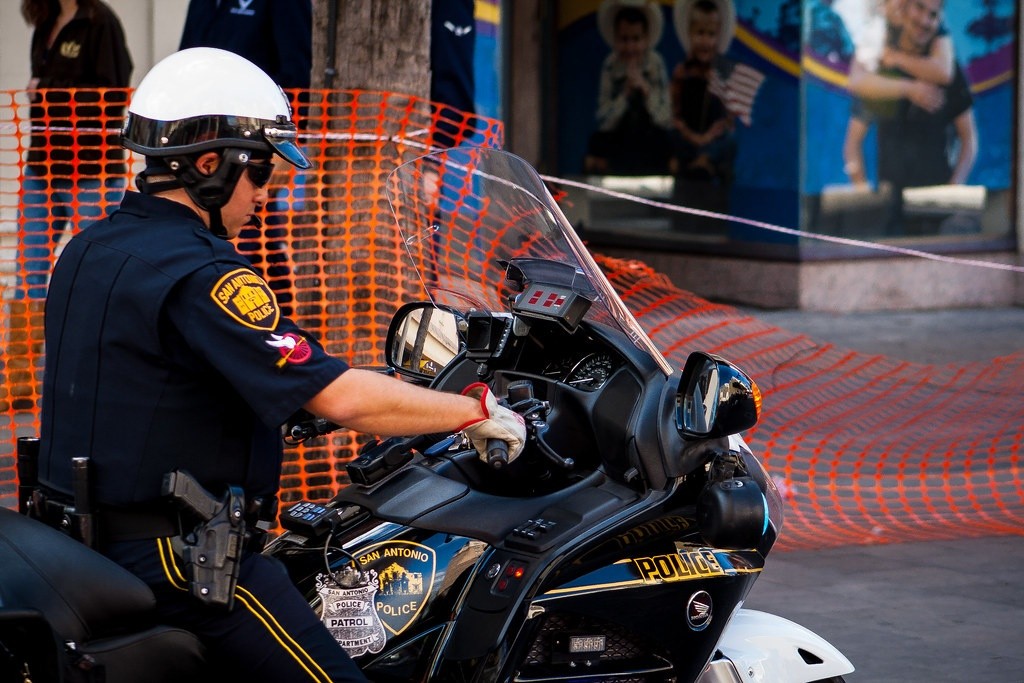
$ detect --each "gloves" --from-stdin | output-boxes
[453,382,526,464]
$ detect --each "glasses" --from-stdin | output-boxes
[246,161,273,187]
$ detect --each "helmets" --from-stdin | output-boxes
[119,46,311,169]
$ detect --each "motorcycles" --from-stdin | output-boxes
[1,159,863,683]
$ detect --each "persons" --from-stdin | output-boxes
[584,1,981,232]
[34,46,528,683]
[12,0,477,432]
